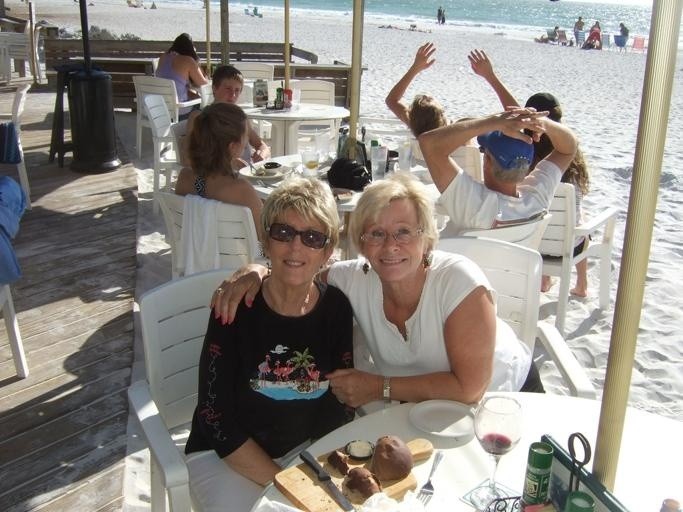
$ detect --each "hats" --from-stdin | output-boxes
[478,129,534,169]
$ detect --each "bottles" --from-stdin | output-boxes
[253,78,269,106]
[524,440,596,511]
[276,88,292,105]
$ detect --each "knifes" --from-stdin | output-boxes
[299,451,358,512]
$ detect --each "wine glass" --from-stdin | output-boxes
[471,396,522,509]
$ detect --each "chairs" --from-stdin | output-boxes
[410,138,483,184]
[156,193,259,279]
[141,94,183,197]
[2,84,30,208]
[132,75,200,158]
[350,235,595,417]
[197,77,254,108]
[458,214,552,251]
[630,37,645,54]
[128,270,233,510]
[200,82,272,139]
[282,80,342,148]
[170,118,187,166]
[2,178,29,379]
[357,115,410,147]
[614,35,628,54]
[539,182,618,335]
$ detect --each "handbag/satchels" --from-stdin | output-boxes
[327,157,371,191]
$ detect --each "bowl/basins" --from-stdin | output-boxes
[263,167,280,174]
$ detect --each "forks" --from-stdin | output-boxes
[415,450,444,506]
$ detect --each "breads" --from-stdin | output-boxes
[328,435,413,502]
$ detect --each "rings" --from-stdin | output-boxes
[216,287,225,296]
[338,400,345,404]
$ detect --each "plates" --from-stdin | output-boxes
[409,399,478,438]
[239,164,292,179]
[332,188,357,202]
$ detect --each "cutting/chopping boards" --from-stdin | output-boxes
[271,438,434,512]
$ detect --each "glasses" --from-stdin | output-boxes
[266,223,327,249]
[361,229,420,245]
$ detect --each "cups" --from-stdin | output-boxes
[302,147,319,177]
[370,146,387,179]
[398,146,412,171]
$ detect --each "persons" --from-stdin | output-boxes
[524,92,587,297]
[418,103,579,240]
[173,101,268,265]
[183,178,352,511]
[441,9,448,24]
[207,177,545,408]
[385,41,522,146]
[156,31,208,121]
[437,6,442,24]
[184,63,270,175]
[537,15,630,51]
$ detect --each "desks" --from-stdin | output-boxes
[239,151,472,259]
[244,103,349,157]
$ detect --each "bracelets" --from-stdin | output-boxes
[383,375,391,404]
